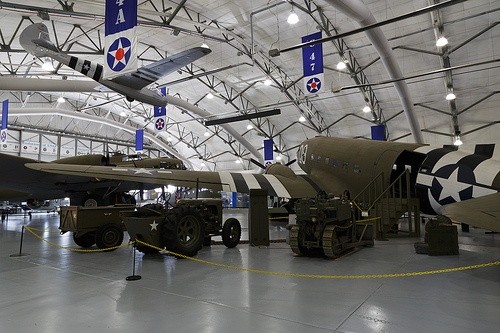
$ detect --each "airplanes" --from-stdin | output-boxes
[24,134,500,234]
[0,150,186,208]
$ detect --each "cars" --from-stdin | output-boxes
[0,203,22,214]
